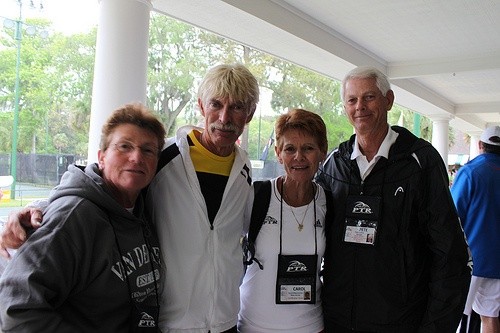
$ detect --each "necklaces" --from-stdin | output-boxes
[285,187,308,231]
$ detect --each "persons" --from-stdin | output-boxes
[1,63,260,332]
[0,107,165,332]
[314,67,471,333]
[237,110,334,333]
[450,126,500,333]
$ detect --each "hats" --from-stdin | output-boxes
[480,125,500,146]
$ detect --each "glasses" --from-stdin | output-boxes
[108,140,158,159]
